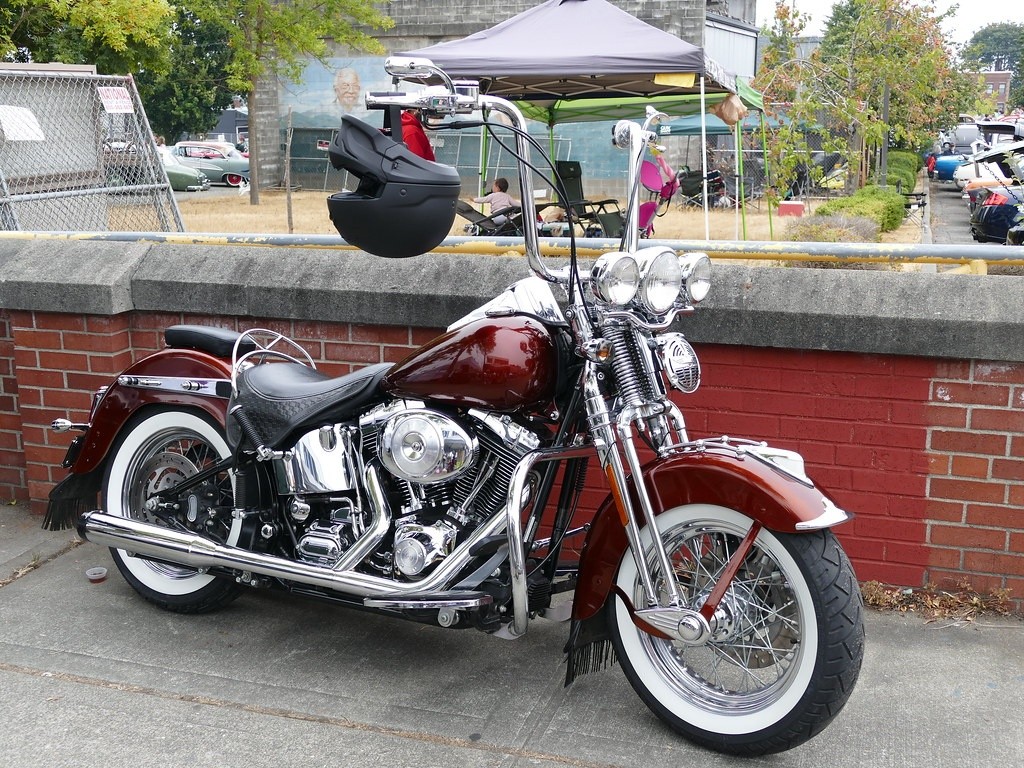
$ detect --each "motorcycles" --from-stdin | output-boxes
[41,55,869,758]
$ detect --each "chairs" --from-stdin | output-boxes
[554,160,622,238]
[449,199,544,240]
[896,181,926,229]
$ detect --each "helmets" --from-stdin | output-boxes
[326,115,463,259]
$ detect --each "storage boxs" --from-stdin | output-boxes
[776,201,807,216]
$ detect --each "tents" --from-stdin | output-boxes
[394,0,828,242]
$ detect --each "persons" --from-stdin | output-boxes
[400,109,436,161]
[470,178,521,224]
[237,135,249,187]
[153,133,165,146]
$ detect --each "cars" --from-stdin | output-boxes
[100,139,251,195]
[910,110,1024,247]
